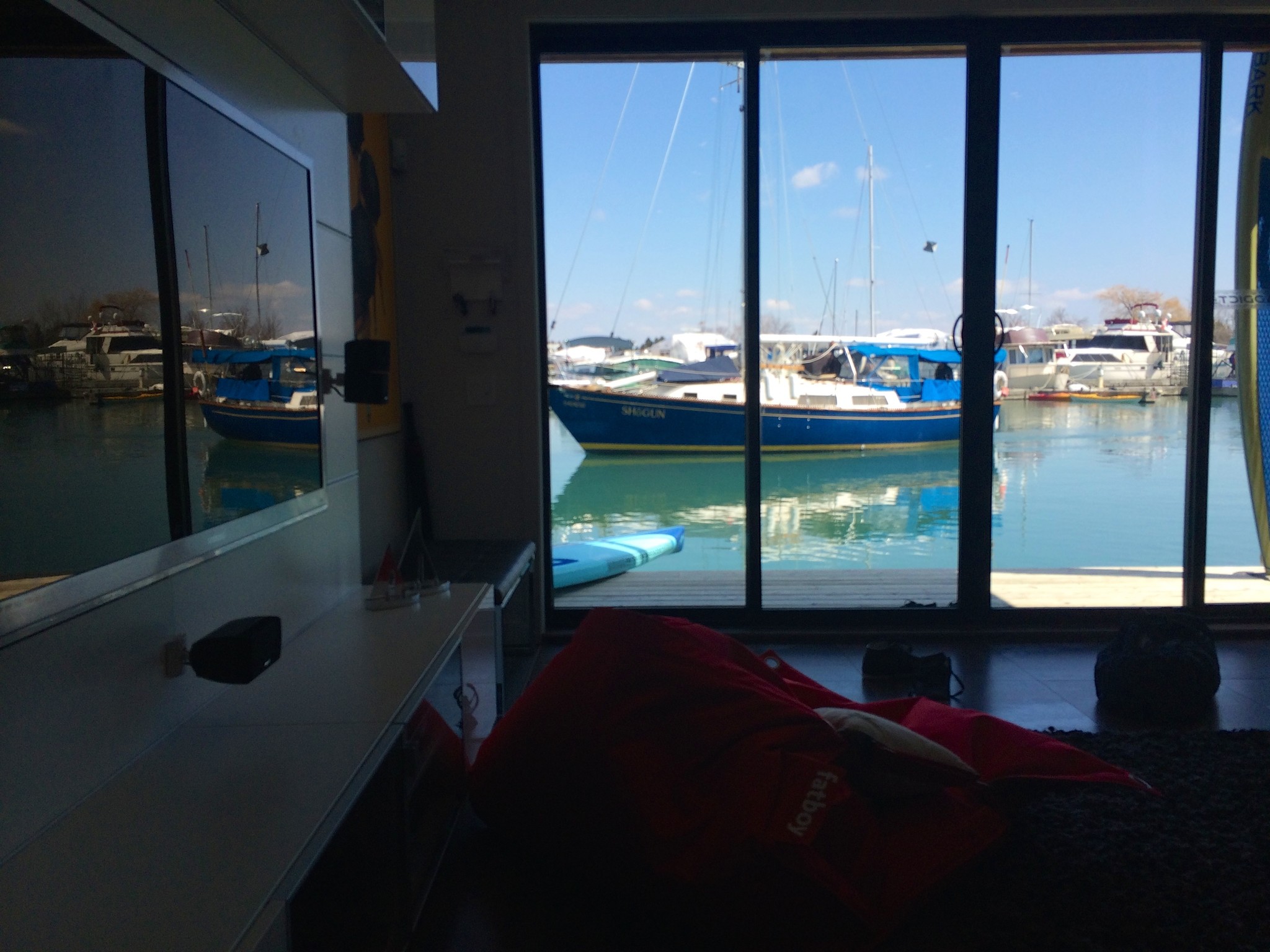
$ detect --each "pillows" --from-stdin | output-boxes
[811,705,982,787]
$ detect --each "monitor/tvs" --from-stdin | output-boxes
[0,0,326,654]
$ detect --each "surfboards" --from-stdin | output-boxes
[551,526,686,589]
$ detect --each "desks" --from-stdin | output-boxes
[0,580,500,952]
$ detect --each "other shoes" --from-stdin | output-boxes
[909,652,965,701]
[862,641,912,679]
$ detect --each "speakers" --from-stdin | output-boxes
[188,616,283,684]
[344,340,390,404]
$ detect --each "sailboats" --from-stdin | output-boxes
[546,61,964,379]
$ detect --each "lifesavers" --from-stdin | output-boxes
[994,370,1009,399]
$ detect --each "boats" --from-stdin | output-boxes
[0,200,320,449]
[548,340,1008,455]
[993,219,1232,389]
